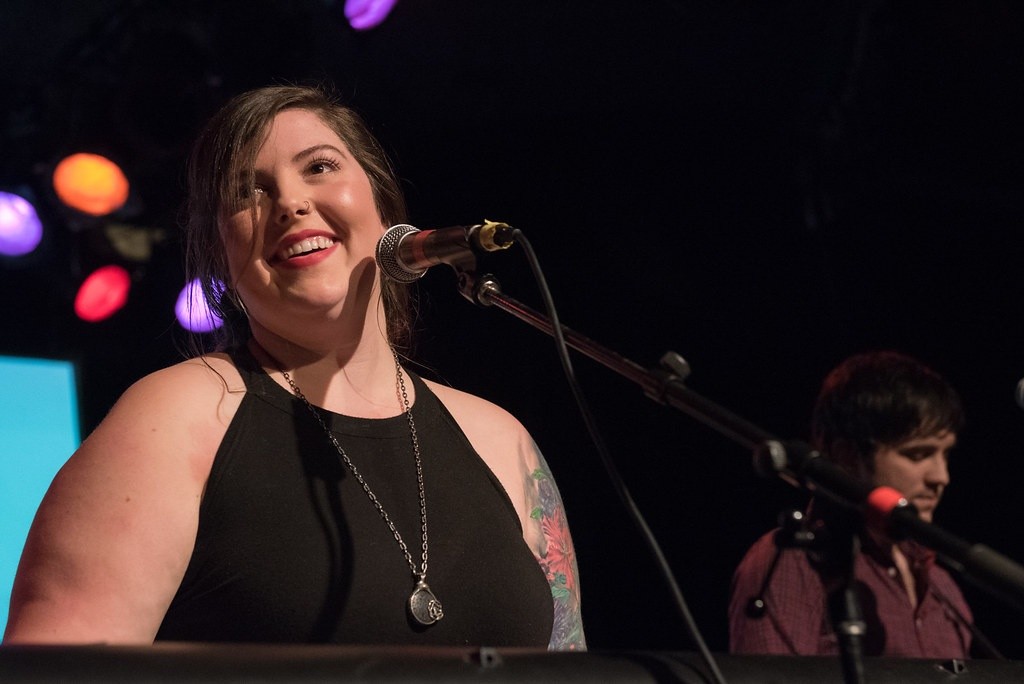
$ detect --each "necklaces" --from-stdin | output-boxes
[262,339,444,628]
[729,351,974,660]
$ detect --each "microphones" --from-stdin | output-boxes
[374,219,518,282]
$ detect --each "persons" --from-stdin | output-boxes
[2,86,590,649]
